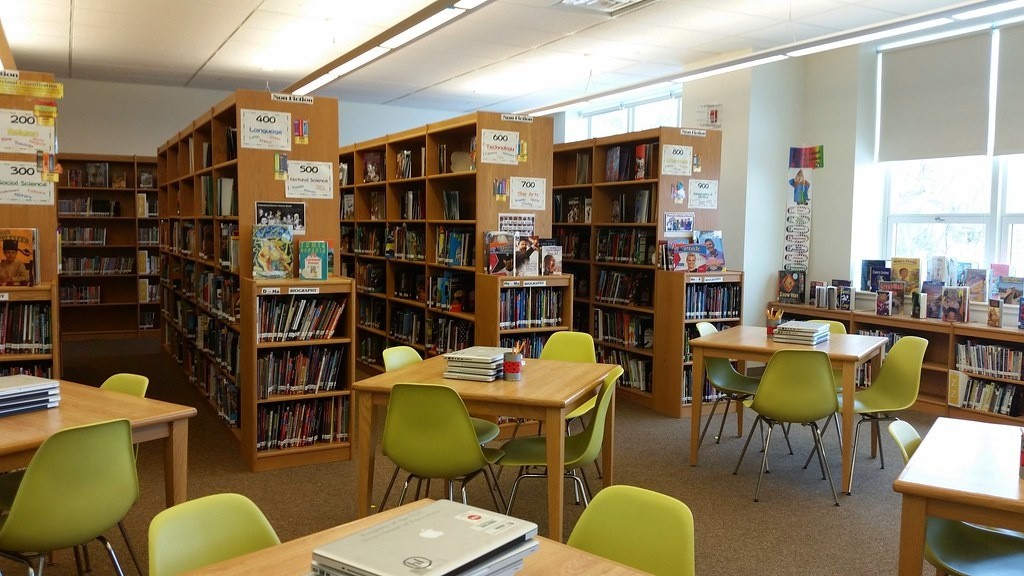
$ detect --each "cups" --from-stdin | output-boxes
[1019,435,1024,478]
[503,352,521,381]
[766,319,781,338]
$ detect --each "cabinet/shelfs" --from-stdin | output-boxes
[0,26,745,471]
[768,300,1024,425]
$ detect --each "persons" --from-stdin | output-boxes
[704,239,725,266]
[0,239,26,281]
[686,252,704,273]
[516,239,538,268]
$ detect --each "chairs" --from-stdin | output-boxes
[1,320,926,576]
[887,421,1024,576]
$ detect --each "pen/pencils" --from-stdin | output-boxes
[515,341,526,355]
[512,348,514,355]
[1020,428,1024,435]
[516,341,519,351]
[767,308,784,320]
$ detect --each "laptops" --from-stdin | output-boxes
[0,374,61,416]
[443,345,525,381]
[311,498,539,576]
[773,321,830,345]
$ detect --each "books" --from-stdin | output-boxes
[483,230,563,277]
[954,339,1024,416]
[682,283,741,405]
[256,295,351,451]
[855,330,903,388]
[252,223,294,279]
[0,226,53,378]
[657,230,725,273]
[299,241,328,280]
[338,137,477,364]
[500,287,562,358]
[58,162,241,430]
[860,257,1024,331]
[809,279,856,311]
[553,143,658,393]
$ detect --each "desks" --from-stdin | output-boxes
[0,379,197,509]
[893,417,1024,576]
[688,326,889,493]
[176,498,655,576]
[351,348,621,543]
